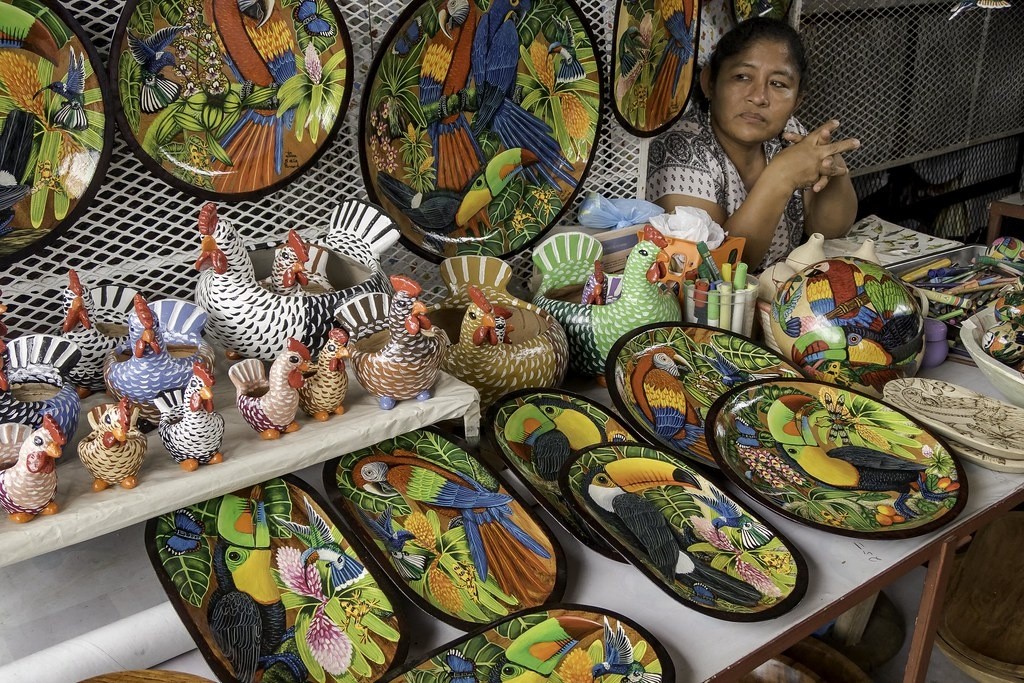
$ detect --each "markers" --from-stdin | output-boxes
[682,241,758,338]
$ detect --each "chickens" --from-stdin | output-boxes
[0,197,683,525]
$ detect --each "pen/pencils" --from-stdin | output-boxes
[897,255,1024,367]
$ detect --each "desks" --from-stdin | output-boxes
[0,188,1024,683]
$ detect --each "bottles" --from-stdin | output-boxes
[787,233,826,278]
[758,262,795,303]
[852,239,881,267]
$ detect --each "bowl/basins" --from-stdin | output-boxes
[959,308,1024,410]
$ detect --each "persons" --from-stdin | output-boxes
[645,17,860,274]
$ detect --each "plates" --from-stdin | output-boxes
[610,0,702,139]
[322,424,568,633]
[557,440,809,623]
[108,0,354,202]
[485,387,644,564]
[358,0,605,265]
[143,474,411,683]
[374,602,677,683]
[940,434,1024,475]
[704,377,969,540]
[0,0,115,269]
[883,376,1024,461]
[604,320,813,483]
